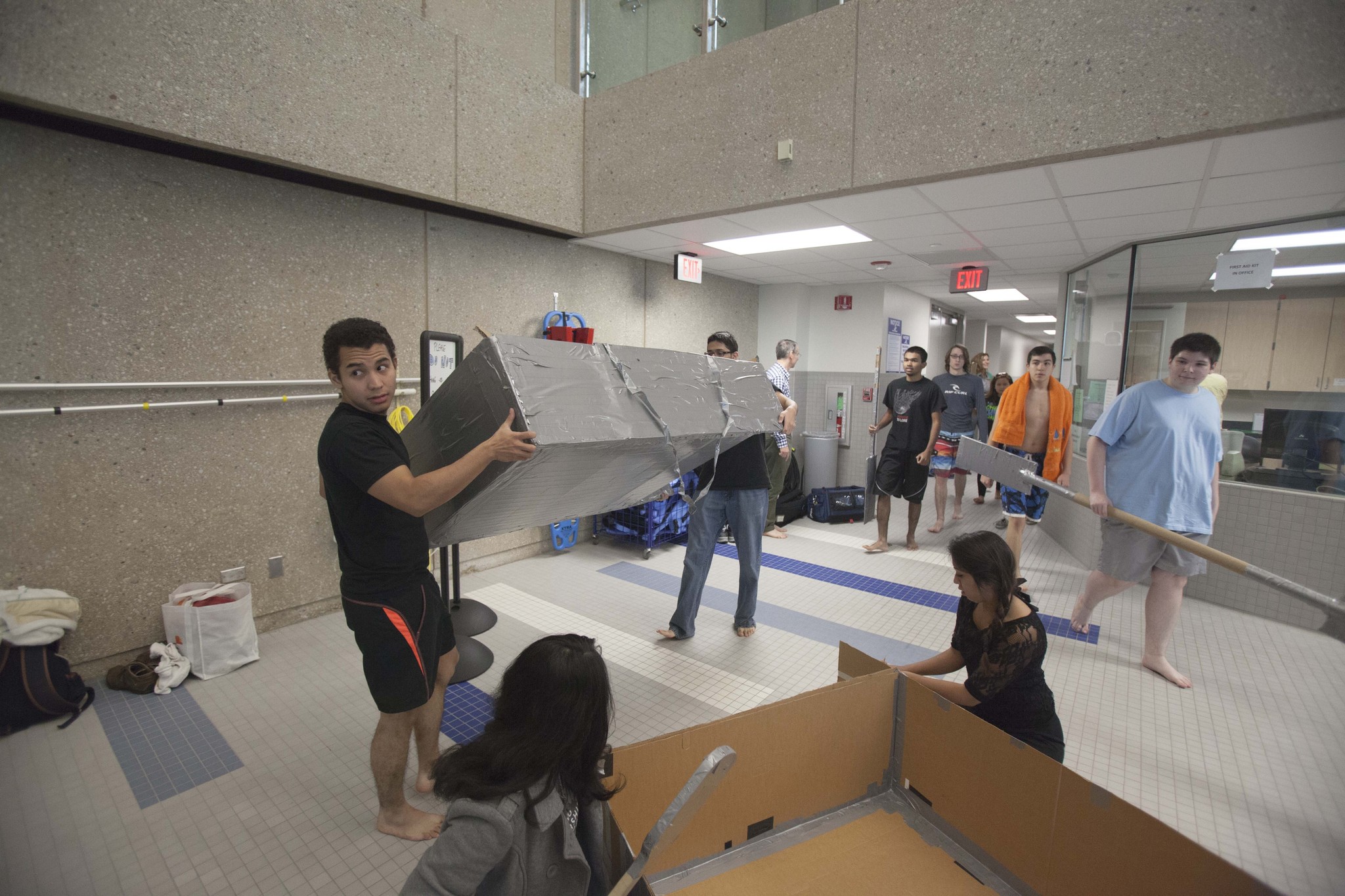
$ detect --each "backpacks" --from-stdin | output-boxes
[0,640,95,736]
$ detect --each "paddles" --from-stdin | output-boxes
[861,347,882,526]
[609,744,740,895]
[953,435,1344,620]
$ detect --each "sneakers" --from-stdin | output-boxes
[106,661,157,693]
[716,525,728,543]
[728,530,735,542]
[138,650,161,671]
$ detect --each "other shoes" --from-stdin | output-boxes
[1025,518,1036,524]
[996,518,1008,529]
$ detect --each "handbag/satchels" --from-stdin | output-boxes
[803,485,865,522]
[161,578,261,681]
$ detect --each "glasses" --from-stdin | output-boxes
[950,354,965,360]
[794,351,802,358]
[704,351,733,358]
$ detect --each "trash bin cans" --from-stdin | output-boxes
[802,429,841,499]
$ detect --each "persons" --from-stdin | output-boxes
[928,344,988,533]
[763,339,802,541]
[971,352,993,396]
[1070,332,1223,688]
[974,372,1014,504]
[980,346,1074,590]
[881,531,1065,765]
[862,345,948,552]
[657,333,799,640]
[397,633,654,896]
[316,318,536,840]
